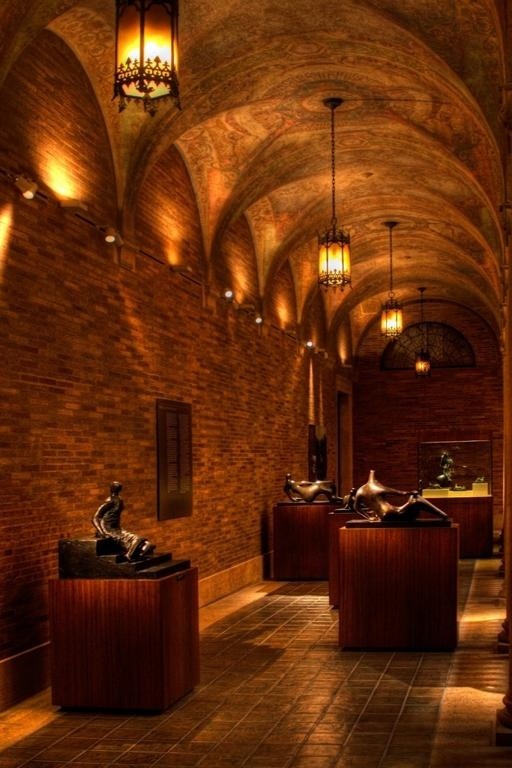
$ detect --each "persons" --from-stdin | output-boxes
[283,472,344,505]
[353,469,449,524]
[92,481,152,563]
[436,449,457,486]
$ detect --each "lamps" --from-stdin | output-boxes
[377,217,407,339]
[108,0,185,116]
[103,222,125,245]
[312,98,358,291]
[14,168,40,203]
[415,285,433,376]
